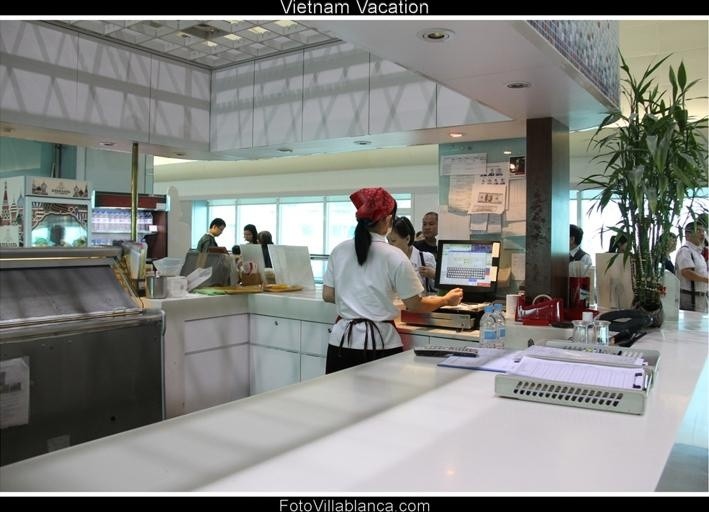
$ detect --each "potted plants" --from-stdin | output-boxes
[574,46,708,328]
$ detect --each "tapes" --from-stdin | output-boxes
[532,294,552,305]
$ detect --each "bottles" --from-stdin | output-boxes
[479,304,506,353]
[92,209,153,231]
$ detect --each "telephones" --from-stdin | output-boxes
[599,310,653,332]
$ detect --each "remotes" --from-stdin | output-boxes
[414,344,481,358]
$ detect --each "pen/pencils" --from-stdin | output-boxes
[644,374,648,391]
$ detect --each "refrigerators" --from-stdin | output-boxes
[1,177,92,248]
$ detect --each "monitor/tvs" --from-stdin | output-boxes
[434,240,501,303]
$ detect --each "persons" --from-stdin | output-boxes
[197,218,227,252]
[609,213,709,313]
[323,187,445,376]
[511,157,521,175]
[49,225,69,247]
[259,231,276,267]
[569,224,593,275]
[243,224,259,244]
